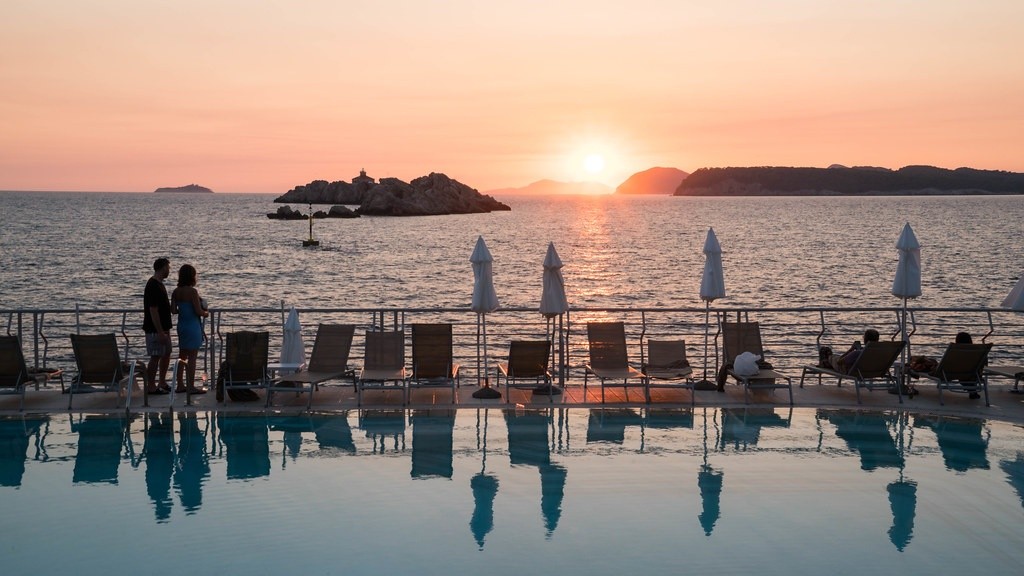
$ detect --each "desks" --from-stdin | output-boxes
[983,364,1024,394]
[266,416,313,433]
[267,362,307,398]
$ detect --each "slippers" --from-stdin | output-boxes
[148,387,169,394]
[176,386,187,393]
[188,388,207,394]
[157,385,172,392]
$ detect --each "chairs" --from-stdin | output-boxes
[496,339,554,404]
[359,409,407,436]
[221,330,274,409]
[0,335,66,410]
[0,413,52,487]
[266,323,358,408]
[69,332,150,410]
[307,409,357,455]
[70,410,150,484]
[584,322,650,403]
[358,330,410,408]
[799,340,904,404]
[721,322,794,406]
[641,339,696,405]
[646,408,695,430]
[587,408,644,443]
[215,412,270,479]
[720,409,793,445]
[816,409,905,469]
[406,324,455,406]
[502,408,551,468]
[905,341,994,408]
[909,413,990,471]
[409,409,459,477]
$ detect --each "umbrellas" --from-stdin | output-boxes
[693,227,725,390]
[533,241,568,395]
[889,222,921,396]
[469,236,501,399]
[279,307,305,377]
[1001,275,1024,312]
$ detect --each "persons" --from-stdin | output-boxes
[171,264,209,395]
[142,258,172,395]
[816,329,879,374]
[910,332,973,372]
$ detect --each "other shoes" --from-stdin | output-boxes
[820,346,832,367]
[969,393,980,399]
